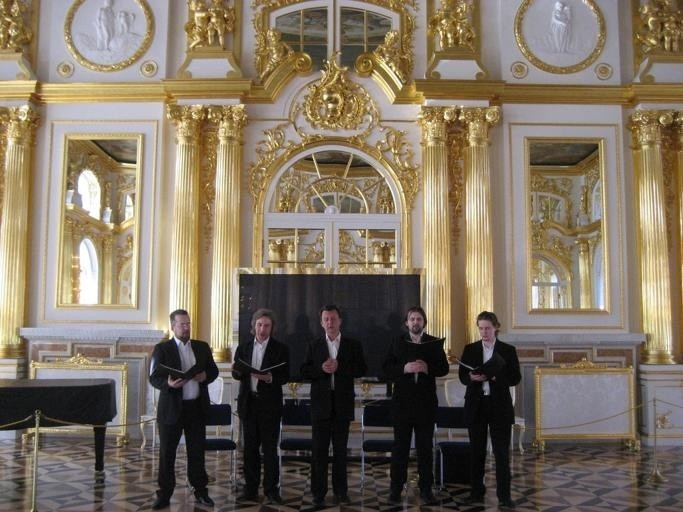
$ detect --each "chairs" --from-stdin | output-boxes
[205,399,484,492]
[138,387,159,449]
[207,377,224,454]
[444,378,526,455]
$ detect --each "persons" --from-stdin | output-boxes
[639,0,683,52]
[434,0,455,48]
[0,0,34,50]
[188,1,210,49]
[119,12,129,32]
[375,30,408,83]
[299,302,369,504]
[550,0,572,53]
[206,1,230,50]
[230,306,291,506]
[257,27,295,79]
[381,241,392,269]
[95,0,115,51]
[149,308,219,510]
[277,239,287,267]
[456,0,476,47]
[457,311,522,509]
[377,306,450,505]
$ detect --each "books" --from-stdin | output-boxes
[231,358,289,377]
[150,363,207,382]
[453,352,506,382]
[399,336,445,363]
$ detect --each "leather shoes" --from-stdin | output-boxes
[464,495,515,508]
[312,496,325,506]
[152,497,171,510]
[198,494,215,507]
[235,491,259,502]
[267,494,288,506]
[334,495,352,505]
[387,491,403,505]
[420,492,439,506]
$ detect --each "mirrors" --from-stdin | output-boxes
[523,135,611,315]
[54,131,144,311]
